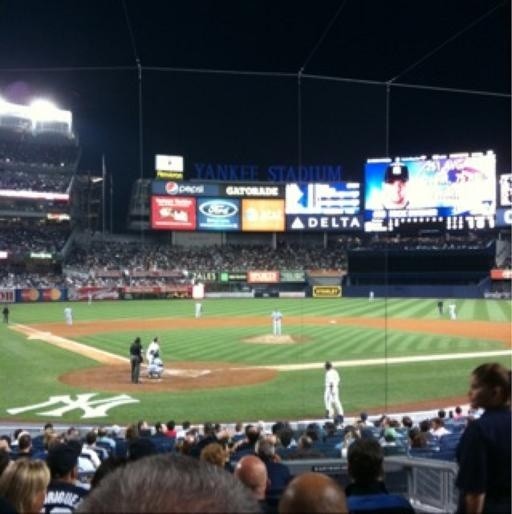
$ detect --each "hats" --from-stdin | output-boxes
[384,164,410,184]
[47,439,82,473]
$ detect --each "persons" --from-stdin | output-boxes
[322,362,345,418]
[436,301,445,315]
[3,304,10,325]
[147,352,164,378]
[130,335,144,383]
[0,126,511,291]
[193,300,204,319]
[64,307,74,327]
[0,401,484,514]
[270,307,285,337]
[448,363,512,512]
[145,335,164,376]
